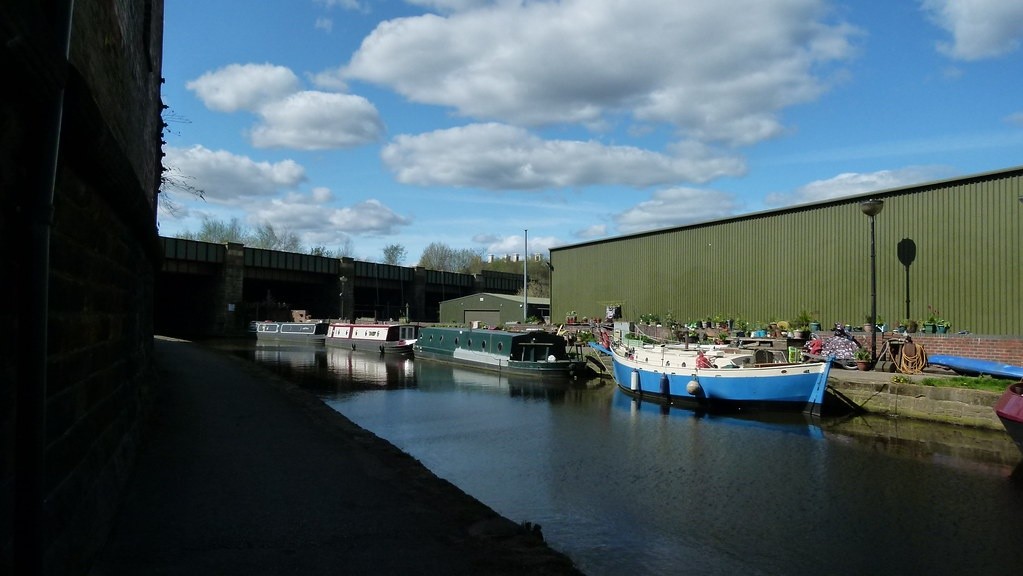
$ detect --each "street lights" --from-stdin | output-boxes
[860,197,885,369]
[339,276,348,324]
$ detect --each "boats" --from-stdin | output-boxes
[255,322,329,345]
[324,323,419,356]
[411,326,586,380]
[993,382,1023,454]
[928,354,1023,381]
[610,332,836,417]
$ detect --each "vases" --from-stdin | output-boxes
[898,326,906,333]
[923,323,936,334]
[649,321,657,326]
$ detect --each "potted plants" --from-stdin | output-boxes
[793,325,812,339]
[787,328,794,337]
[935,320,952,334]
[639,313,648,325]
[853,347,871,371]
[788,310,814,330]
[663,312,782,345]
[809,319,821,332]
[875,313,886,332]
[863,314,872,333]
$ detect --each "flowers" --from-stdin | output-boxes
[894,320,906,327]
[924,304,938,324]
[648,313,659,322]
